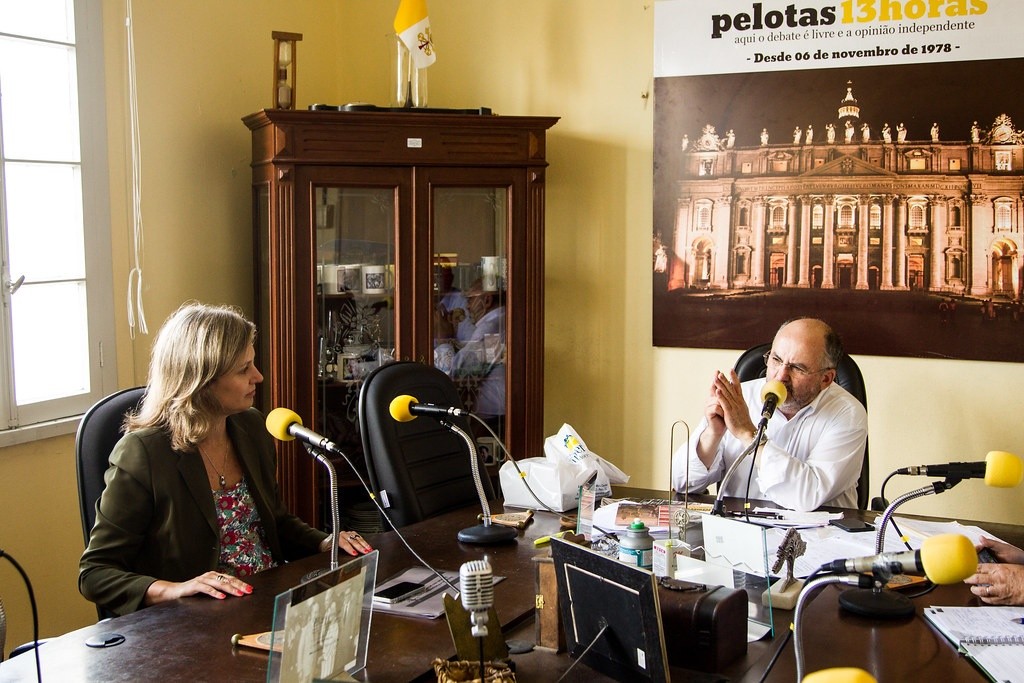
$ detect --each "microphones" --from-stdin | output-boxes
[897,451,1024,488]
[447,308,466,322]
[363,297,394,310]
[460,561,494,636]
[821,535,978,585]
[265,407,342,454]
[760,379,787,419]
[388,395,469,422]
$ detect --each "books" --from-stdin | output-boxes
[591,496,1011,582]
[920,605,1024,683]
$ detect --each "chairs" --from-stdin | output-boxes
[357,360,497,532]
[0,548,57,663]
[76,384,147,621]
[734,343,890,513]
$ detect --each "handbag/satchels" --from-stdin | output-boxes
[501,424,631,513]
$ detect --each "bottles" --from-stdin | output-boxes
[618,517,656,570]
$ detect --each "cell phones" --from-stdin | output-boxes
[373,582,425,603]
[829,518,875,532]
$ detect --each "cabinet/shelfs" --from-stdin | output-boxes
[242,109,562,562]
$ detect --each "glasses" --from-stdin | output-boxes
[762,349,833,377]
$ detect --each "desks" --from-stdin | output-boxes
[0,487,1024,683]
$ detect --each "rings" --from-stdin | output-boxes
[347,534,360,541]
[217,575,229,583]
[986,586,990,597]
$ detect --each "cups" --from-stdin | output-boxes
[337,353,360,382]
[433,256,505,291]
[316,264,395,294]
[477,437,506,466]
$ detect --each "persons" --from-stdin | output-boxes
[793,125,814,145]
[939,297,1024,324]
[435,251,510,438]
[283,583,363,683]
[931,123,940,141]
[77,300,373,618]
[971,121,981,143]
[826,121,870,143]
[726,129,736,149]
[760,128,768,145]
[965,536,1024,605]
[883,122,907,143]
[672,316,868,513]
[655,245,668,273]
[682,134,688,151]
[316,282,377,419]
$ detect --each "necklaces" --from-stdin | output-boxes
[197,440,232,486]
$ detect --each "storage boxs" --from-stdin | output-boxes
[653,538,690,578]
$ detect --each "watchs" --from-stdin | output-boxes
[743,430,768,455]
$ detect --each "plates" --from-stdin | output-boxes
[560,514,578,527]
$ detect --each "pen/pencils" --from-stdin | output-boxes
[534,529,573,545]
[729,510,784,520]
